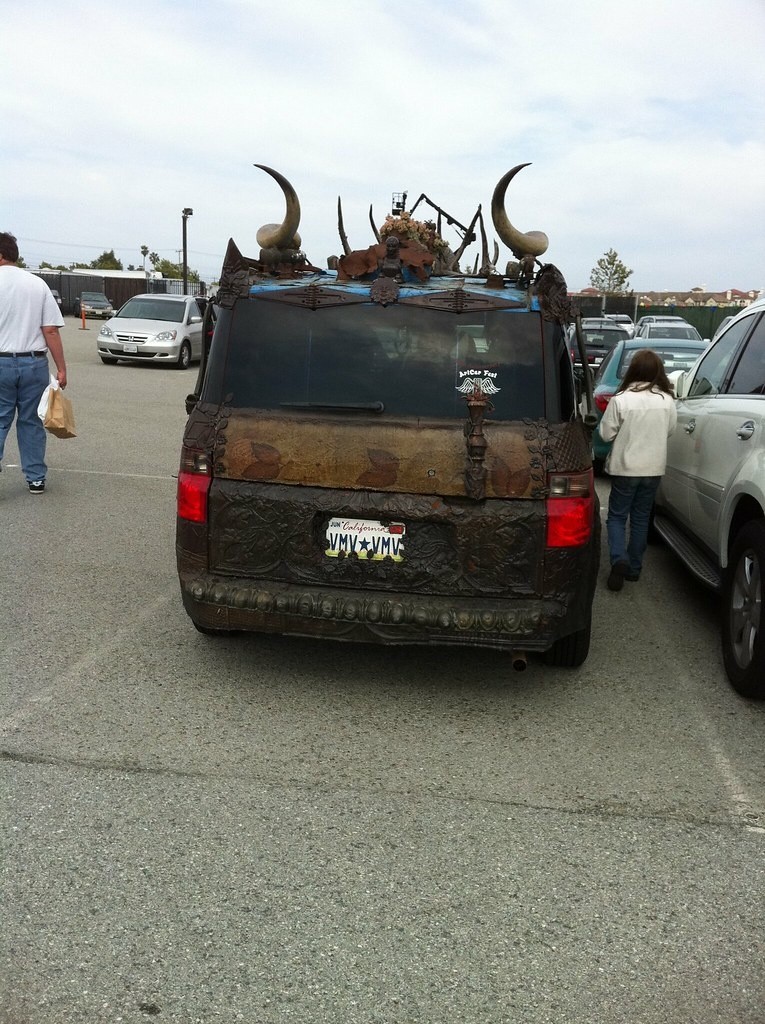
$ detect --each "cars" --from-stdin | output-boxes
[567,313,736,469]
[51,289,65,317]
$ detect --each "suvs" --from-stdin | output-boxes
[647,299,765,706]
[173,157,603,670]
[96,293,220,370]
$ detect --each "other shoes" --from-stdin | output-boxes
[607,560,626,591]
[625,573,639,581]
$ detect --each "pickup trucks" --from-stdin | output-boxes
[73,292,114,320]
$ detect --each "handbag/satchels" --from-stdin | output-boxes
[36,373,77,438]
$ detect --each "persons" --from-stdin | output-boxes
[0,231,67,495]
[596,347,679,592]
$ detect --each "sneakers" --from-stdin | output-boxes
[27,479,45,494]
